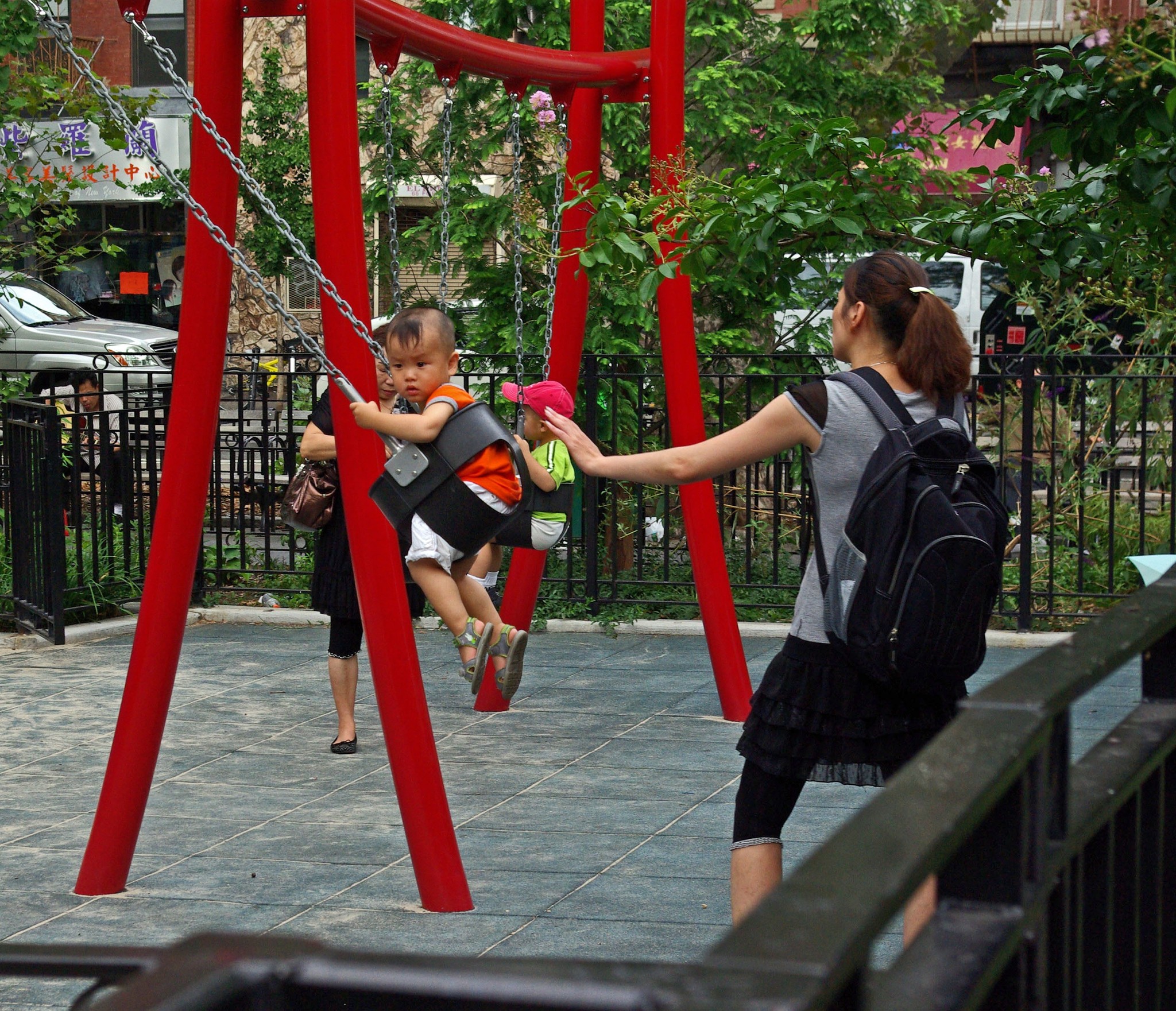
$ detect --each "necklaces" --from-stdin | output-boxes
[867,360,898,367]
[380,395,396,412]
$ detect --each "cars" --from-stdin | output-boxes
[0,268,179,408]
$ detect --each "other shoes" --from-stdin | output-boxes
[113,514,124,532]
[330,732,357,755]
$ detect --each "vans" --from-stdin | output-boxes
[765,248,1018,390]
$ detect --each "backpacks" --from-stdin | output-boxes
[810,366,1012,692]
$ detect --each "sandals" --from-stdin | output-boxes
[451,617,494,694]
[487,624,529,700]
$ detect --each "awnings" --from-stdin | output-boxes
[365,174,505,201]
[750,106,1033,205]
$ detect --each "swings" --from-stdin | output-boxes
[494,89,576,550]
[22,0,534,565]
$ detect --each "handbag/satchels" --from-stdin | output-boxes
[279,459,339,532]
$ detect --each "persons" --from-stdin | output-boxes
[73,272,99,301]
[162,278,182,307]
[463,380,574,592]
[300,316,401,756]
[542,248,970,957]
[384,304,529,700]
[39,366,130,534]
[171,256,186,298]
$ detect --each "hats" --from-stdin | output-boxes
[502,380,575,427]
